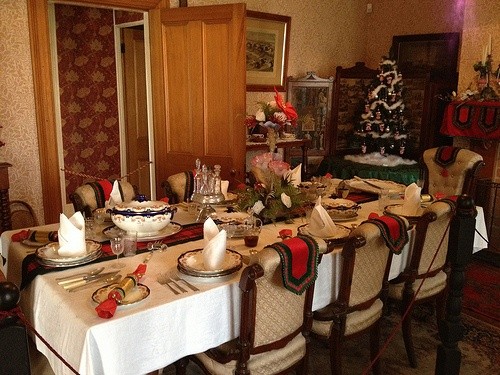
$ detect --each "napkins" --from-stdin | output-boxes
[203,217,227,270]
[309,195,338,236]
[105,180,123,206]
[283,163,302,188]
[58,211,87,257]
[221,180,229,200]
[403,182,422,216]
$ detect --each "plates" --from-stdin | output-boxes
[92,281,150,306]
[297,222,351,242]
[178,248,243,278]
[344,177,407,196]
[35,239,104,268]
[103,221,183,241]
[383,203,428,222]
[331,213,358,222]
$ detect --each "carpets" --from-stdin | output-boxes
[285,305,500,375]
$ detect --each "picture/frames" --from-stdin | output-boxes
[391,33,459,80]
[245,10,291,92]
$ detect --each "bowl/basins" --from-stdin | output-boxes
[320,198,359,219]
[110,200,176,236]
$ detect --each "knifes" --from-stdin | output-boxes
[57,267,121,294]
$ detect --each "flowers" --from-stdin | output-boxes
[451,54,500,103]
[229,129,333,227]
[242,86,299,142]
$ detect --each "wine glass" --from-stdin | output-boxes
[95,209,107,236]
[108,238,128,269]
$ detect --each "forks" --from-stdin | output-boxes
[154,271,200,295]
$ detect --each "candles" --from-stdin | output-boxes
[482,35,491,66]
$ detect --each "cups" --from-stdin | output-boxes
[187,202,197,220]
[119,231,137,257]
[243,217,262,247]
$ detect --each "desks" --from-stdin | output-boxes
[0,177,489,375]
[246,139,310,183]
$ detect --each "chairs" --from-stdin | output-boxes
[173,195,461,375]
[70,179,135,219]
[161,169,202,205]
[421,146,486,203]
[0,253,31,375]
